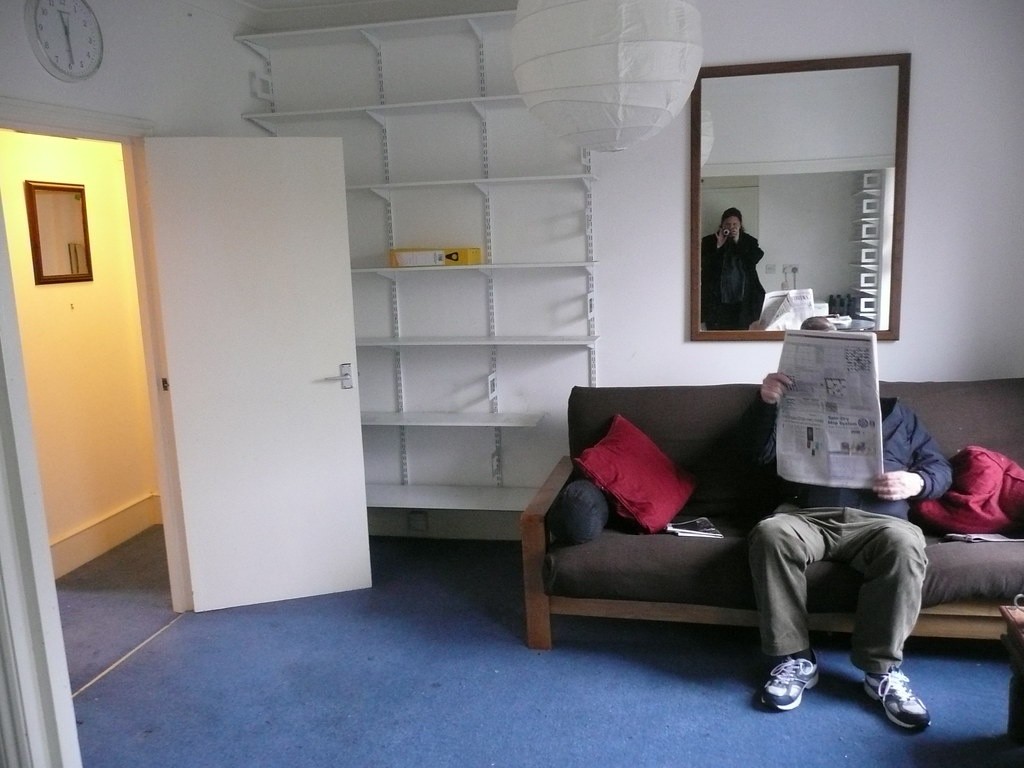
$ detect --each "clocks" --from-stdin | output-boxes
[25,0,103,82]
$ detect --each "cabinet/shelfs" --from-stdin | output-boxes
[849,167,894,328]
[236,8,597,543]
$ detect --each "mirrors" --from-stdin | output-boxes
[691,52,912,342]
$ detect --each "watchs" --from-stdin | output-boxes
[920,479,924,486]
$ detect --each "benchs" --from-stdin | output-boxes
[521,377,1024,650]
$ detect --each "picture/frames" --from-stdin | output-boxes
[23,179,96,285]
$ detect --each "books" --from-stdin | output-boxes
[666,517,724,538]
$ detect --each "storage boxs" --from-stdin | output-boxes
[388,246,482,267]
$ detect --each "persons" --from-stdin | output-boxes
[701,207,765,330]
[740,316,952,730]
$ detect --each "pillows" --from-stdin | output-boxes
[551,480,609,543]
[572,412,695,532]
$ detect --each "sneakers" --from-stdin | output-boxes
[863,663,932,728]
[761,648,819,710]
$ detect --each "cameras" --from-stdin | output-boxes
[721,227,734,237]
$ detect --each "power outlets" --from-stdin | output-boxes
[765,264,776,273]
[783,265,799,272]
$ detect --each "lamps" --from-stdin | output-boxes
[514,0,702,152]
